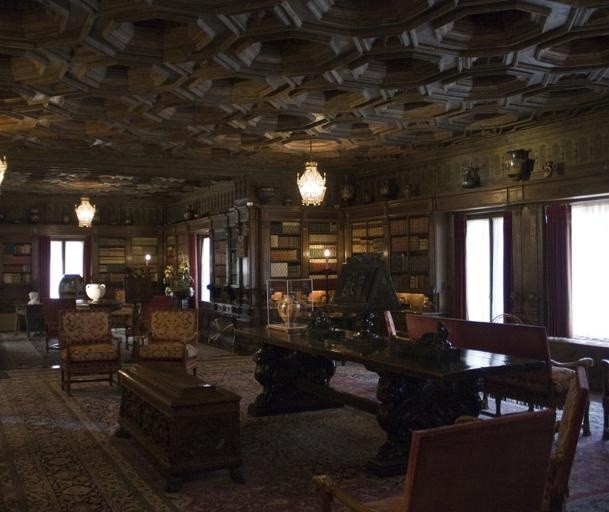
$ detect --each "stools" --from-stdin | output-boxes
[11,303,43,338]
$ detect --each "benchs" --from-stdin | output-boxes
[403,312,594,436]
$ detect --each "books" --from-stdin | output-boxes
[98,236,159,282]
[1,243,32,283]
[270,216,429,296]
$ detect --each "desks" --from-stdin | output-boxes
[78,300,122,312]
[234,326,531,480]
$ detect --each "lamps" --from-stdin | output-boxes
[297,140,327,207]
[75,196,96,228]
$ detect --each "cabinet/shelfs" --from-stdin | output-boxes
[91,224,164,284]
[344,194,434,293]
[261,206,344,302]
[0,240,33,314]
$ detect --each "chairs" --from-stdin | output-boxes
[134,307,199,376]
[384,311,409,341]
[310,409,556,512]
[598,359,609,440]
[454,365,591,512]
[57,307,122,396]
[126,295,173,351]
[43,297,76,354]
